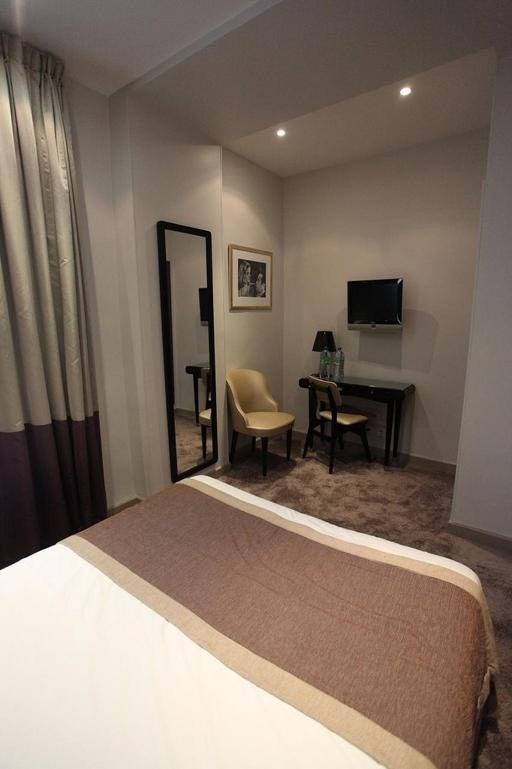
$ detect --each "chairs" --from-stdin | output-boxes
[227,367,295,476]
[198,368,212,458]
[299,376,372,474]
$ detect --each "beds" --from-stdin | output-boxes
[0,475,481,768]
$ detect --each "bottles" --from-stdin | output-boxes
[319,347,331,379]
[332,348,344,379]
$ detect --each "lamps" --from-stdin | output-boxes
[313,328,336,353]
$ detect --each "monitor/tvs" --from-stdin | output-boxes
[199,288,208,326]
[347,278,403,331]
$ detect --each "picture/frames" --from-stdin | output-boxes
[228,242,272,312]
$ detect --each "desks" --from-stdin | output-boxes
[298,373,415,466]
[185,363,209,423]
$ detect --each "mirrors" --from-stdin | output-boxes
[156,219,218,482]
